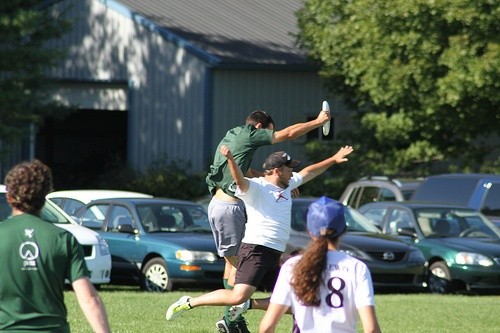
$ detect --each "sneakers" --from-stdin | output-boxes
[216,314,250,333]
[228,298,250,322]
[166,296,192,321]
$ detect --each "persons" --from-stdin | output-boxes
[0,160,113,333]
[166,111,354,333]
[259,197,384,333]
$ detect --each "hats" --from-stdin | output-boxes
[306,196,346,238]
[263,151,301,170]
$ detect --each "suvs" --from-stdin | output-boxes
[336,175,422,212]
[411,173,500,237]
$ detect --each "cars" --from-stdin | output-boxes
[194,199,426,293]
[72,196,226,293]
[45,190,177,232]
[0,184,113,290]
[358,202,500,297]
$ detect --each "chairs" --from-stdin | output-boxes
[114,216,131,229]
[396,222,409,235]
[434,220,450,234]
[152,215,176,231]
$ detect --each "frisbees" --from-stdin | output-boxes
[322,100,331,135]
